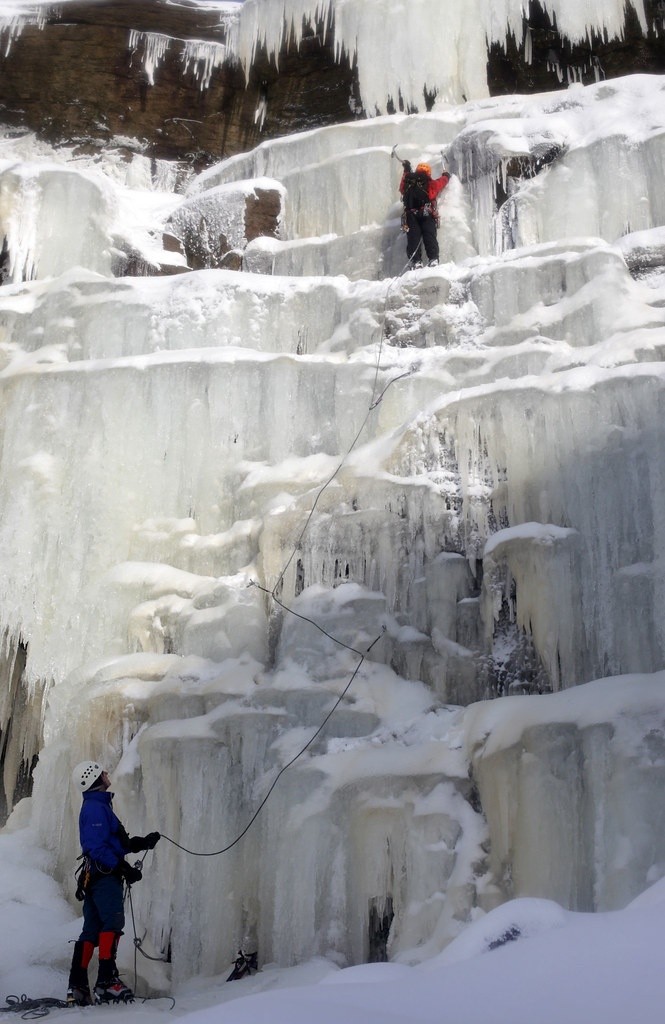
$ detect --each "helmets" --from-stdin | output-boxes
[415,163,431,176]
[72,761,102,792]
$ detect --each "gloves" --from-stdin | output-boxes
[130,831,160,853]
[401,160,412,171]
[441,172,451,179]
[114,860,141,884]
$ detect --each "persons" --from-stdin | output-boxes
[66,761,161,1006]
[399,160,453,267]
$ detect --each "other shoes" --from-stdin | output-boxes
[410,261,424,271]
[426,259,439,267]
[94,980,131,998]
[66,985,93,1005]
